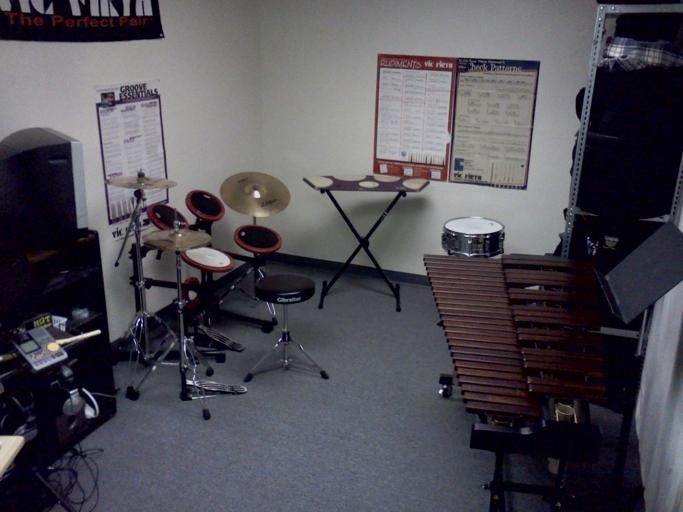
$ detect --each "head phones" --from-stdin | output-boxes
[59,364,86,418]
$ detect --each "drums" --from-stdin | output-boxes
[186,189,225,220]
[442,216,504,259]
[234,224,282,254]
[181,248,233,271]
[148,202,189,230]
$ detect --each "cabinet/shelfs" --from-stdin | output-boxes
[548,0,683,369]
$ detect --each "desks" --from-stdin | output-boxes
[301,173,429,312]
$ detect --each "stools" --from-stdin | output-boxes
[243,275,331,385]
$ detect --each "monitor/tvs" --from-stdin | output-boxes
[0,127,89,250]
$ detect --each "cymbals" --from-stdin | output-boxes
[221,172,289,218]
[144,227,211,252]
[106,174,177,189]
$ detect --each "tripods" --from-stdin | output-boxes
[129,252,215,420]
[111,190,198,398]
[219,217,279,326]
